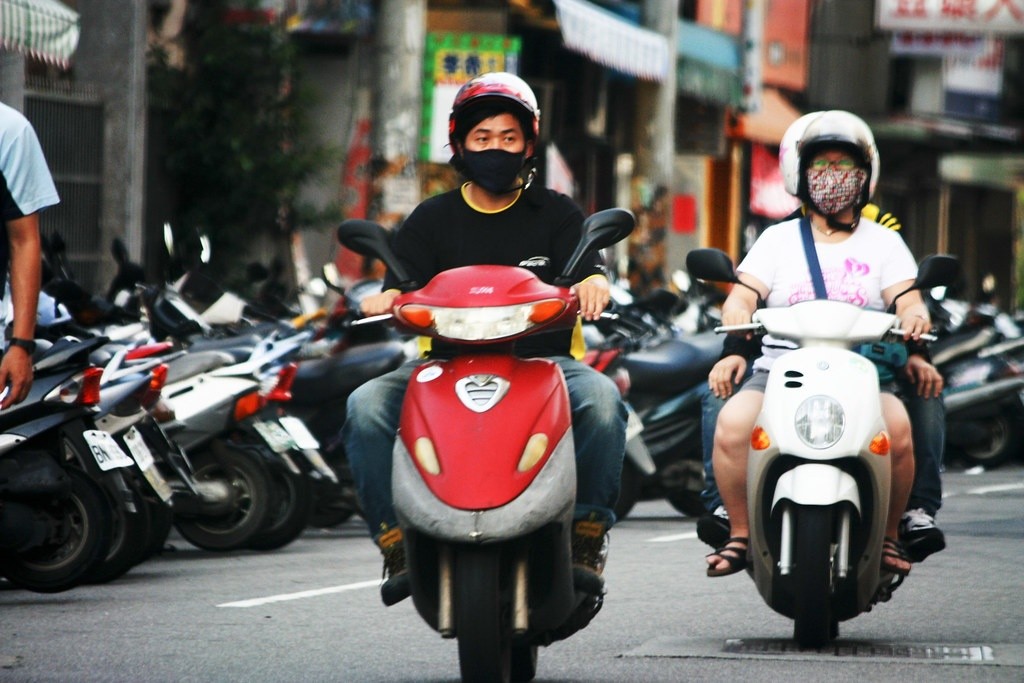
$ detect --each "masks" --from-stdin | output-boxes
[806,170,864,215]
[464,146,526,191]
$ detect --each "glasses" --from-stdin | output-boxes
[813,158,861,169]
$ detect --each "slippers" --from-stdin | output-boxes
[883,536,911,576]
[706,537,750,576]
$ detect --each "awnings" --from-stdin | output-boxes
[509,0,672,82]
[674,20,741,106]
[725,91,802,145]
[0,0,81,71]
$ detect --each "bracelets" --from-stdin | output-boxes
[9,338,37,354]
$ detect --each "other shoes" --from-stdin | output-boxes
[372,524,408,606]
[571,508,615,591]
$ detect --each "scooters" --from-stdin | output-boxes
[337,208,638,683]
[686,248,965,650]
[0,221,1024,594]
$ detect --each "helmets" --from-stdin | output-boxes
[779,110,880,201]
[448,73,539,155]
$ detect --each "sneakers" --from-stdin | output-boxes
[899,510,946,557]
[697,515,731,549]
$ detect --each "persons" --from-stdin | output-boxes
[343,68,632,609]
[0,100,61,412]
[696,109,943,567]
[704,109,937,581]
